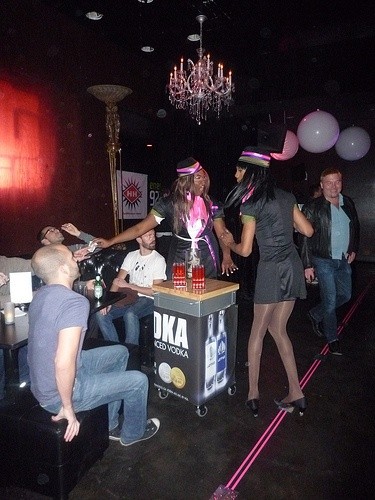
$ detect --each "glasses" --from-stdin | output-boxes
[42,227,60,239]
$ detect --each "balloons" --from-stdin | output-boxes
[270,108,371,161]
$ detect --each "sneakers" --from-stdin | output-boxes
[120,418,160,447]
[108,425,122,441]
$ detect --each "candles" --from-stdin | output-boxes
[4,302,15,325]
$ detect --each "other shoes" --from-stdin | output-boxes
[328,340,343,355]
[307,311,324,337]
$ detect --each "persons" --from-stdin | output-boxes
[93,157,238,280]
[37,223,108,338]
[221,146,316,416]
[96,229,168,346]
[299,168,360,357]
[31,245,161,448]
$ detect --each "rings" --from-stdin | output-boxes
[231,265,233,266]
[221,236,224,238]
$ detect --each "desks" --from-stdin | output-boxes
[0,289,126,385]
[145,279,239,416]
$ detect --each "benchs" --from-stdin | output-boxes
[0,339,144,500]
[79,236,174,365]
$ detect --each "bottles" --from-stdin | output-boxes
[94,274,103,300]
[205,313,216,398]
[217,310,227,390]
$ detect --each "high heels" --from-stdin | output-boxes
[245,398,259,417]
[274,395,306,416]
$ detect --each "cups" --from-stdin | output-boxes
[74,281,87,297]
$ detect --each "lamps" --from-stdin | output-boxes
[9,272,33,311]
[167,13,233,125]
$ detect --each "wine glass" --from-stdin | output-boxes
[55,222,73,234]
